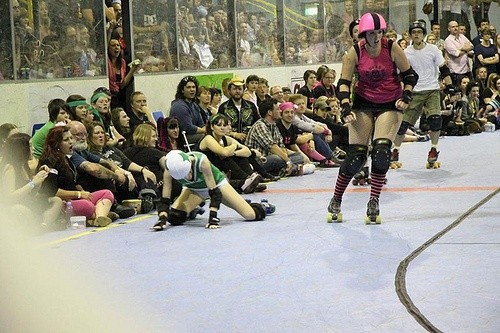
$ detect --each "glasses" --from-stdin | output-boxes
[72,132,89,139]
[183,76,196,82]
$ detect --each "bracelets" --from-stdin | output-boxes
[233,132,237,137]
[323,127,330,135]
[77,191,80,198]
[493,55,495,60]
[309,138,314,141]
[233,151,236,156]
[126,171,132,176]
[148,26,151,31]
[141,165,149,174]
[28,181,36,190]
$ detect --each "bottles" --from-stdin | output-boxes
[80,53,87,76]
[64,65,73,78]
[66,202,74,230]
[20,67,31,80]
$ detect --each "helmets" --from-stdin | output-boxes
[358,12,387,38]
[409,20,426,35]
[164,149,191,180]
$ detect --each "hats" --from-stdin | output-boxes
[279,102,294,114]
[228,77,245,85]
[349,19,360,38]
[313,101,331,111]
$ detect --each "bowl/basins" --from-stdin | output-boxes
[484,124,495,132]
[123,199,142,213]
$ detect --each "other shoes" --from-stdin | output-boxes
[87,212,120,227]
[113,204,135,219]
[320,147,347,167]
[140,196,154,214]
[302,164,315,174]
[416,134,430,141]
[241,171,280,194]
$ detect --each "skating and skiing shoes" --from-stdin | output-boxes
[244,198,275,215]
[387,148,402,169]
[189,201,205,219]
[364,197,382,224]
[352,166,387,185]
[327,196,343,223]
[426,147,441,169]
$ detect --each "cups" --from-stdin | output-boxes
[70,215,87,229]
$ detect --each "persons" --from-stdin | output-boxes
[0,0,500,230]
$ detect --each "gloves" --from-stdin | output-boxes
[442,84,455,94]
